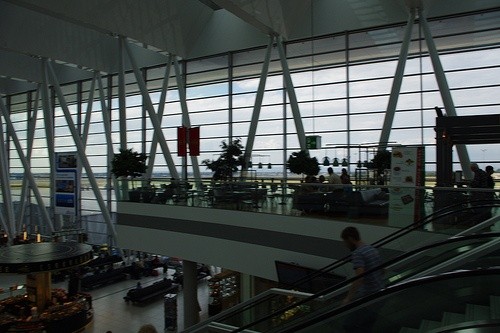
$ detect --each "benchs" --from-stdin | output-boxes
[359,188,389,219]
[81,261,207,306]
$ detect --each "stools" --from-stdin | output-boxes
[241,200,253,212]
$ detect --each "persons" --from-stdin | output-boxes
[470,164,495,188]
[95,247,206,302]
[342,227,383,306]
[306,167,351,184]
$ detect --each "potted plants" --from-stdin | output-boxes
[110,149,148,201]
